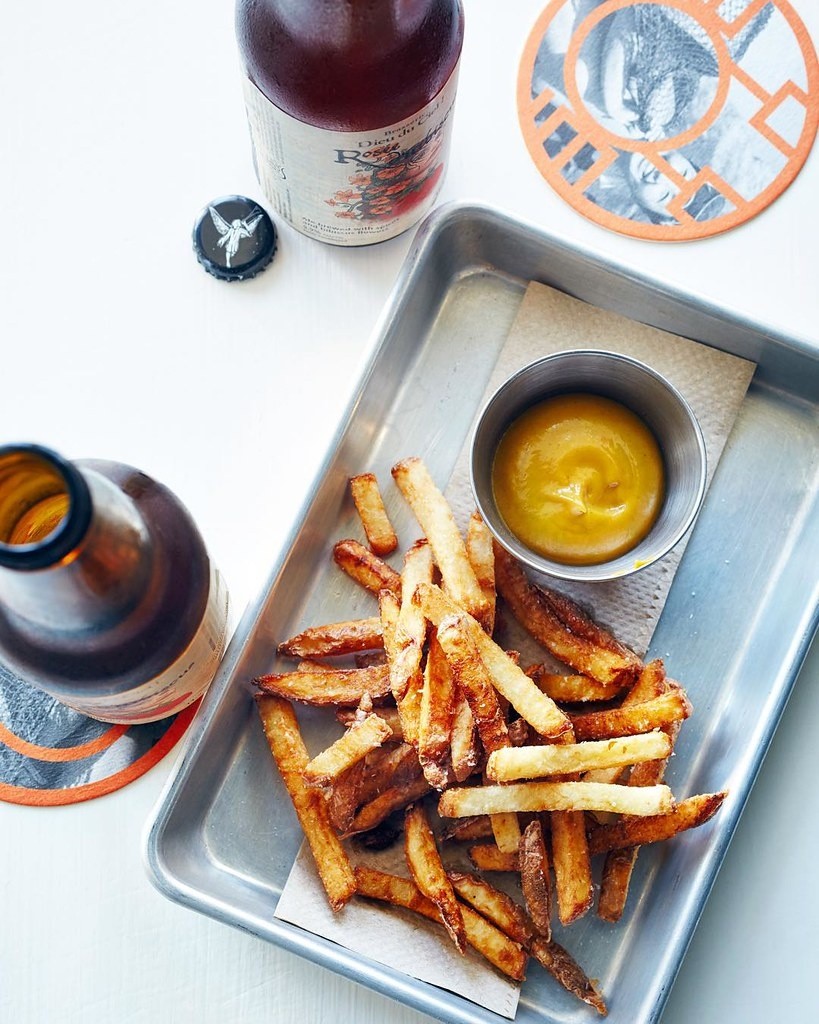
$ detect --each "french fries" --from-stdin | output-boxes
[253,456,729,1017]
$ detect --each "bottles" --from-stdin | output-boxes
[0,440,226,726]
[232,0,466,252]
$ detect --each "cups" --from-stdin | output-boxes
[468,348,709,583]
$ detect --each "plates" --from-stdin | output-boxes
[140,198,819,1024]
